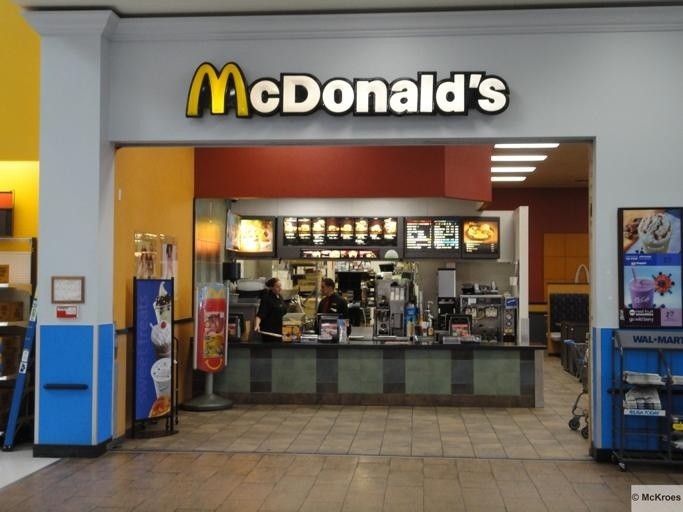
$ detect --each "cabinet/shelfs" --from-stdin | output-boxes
[1,237,38,440]
[607,329,683,472]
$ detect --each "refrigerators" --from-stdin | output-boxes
[132,276,179,434]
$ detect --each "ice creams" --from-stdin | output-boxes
[639,212,672,256]
[150,282,172,398]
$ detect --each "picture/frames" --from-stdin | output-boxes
[50,276,85,303]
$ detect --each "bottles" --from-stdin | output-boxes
[423,321,433,335]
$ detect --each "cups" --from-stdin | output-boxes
[150,299,172,396]
[630,279,656,307]
[638,221,670,252]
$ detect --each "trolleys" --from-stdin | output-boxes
[567,348,590,438]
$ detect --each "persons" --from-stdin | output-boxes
[164,243,176,279]
[254,277,287,342]
[312,277,352,337]
[136,243,156,280]
